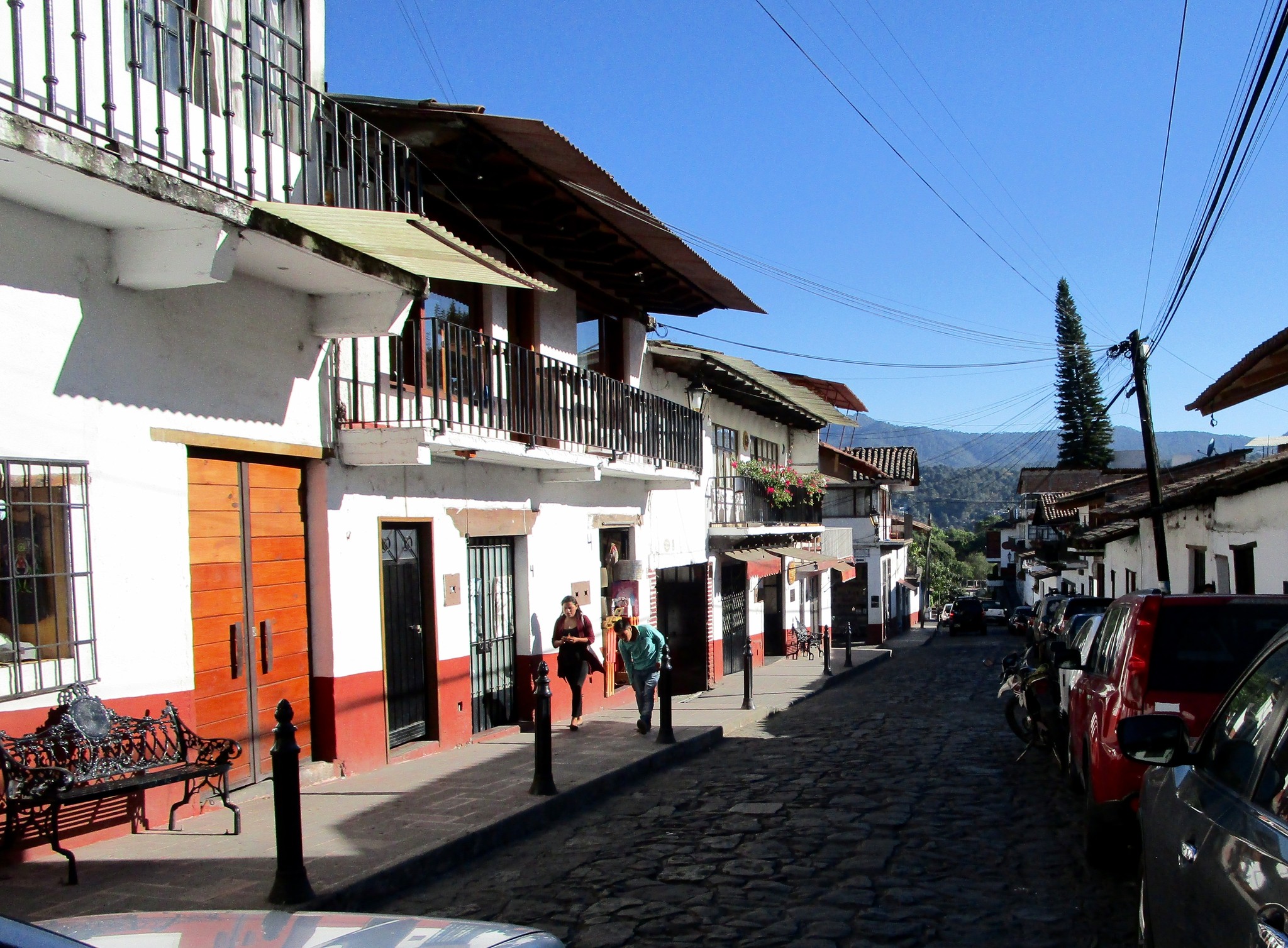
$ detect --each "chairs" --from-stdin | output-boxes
[789,523,822,564]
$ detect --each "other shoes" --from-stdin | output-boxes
[637,723,651,732]
[637,719,647,735]
[577,719,583,725]
[570,725,578,731]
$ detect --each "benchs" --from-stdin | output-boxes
[791,616,824,660]
[0,681,241,883]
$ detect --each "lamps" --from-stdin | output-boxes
[869,506,880,530]
[684,370,712,425]
[1008,552,1014,563]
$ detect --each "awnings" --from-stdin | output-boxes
[897,578,918,595]
[723,548,782,579]
[833,562,856,583]
[765,546,838,570]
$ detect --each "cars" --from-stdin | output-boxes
[940,594,1114,722]
[1115,623,1288,948]
[0,908,570,948]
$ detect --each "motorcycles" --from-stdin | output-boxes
[981,642,1065,773]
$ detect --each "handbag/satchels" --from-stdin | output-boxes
[581,614,604,667]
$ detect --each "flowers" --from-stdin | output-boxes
[732,459,829,508]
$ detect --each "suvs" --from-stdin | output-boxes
[1065,588,1288,818]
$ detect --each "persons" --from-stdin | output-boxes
[551,595,606,730]
[613,619,666,734]
